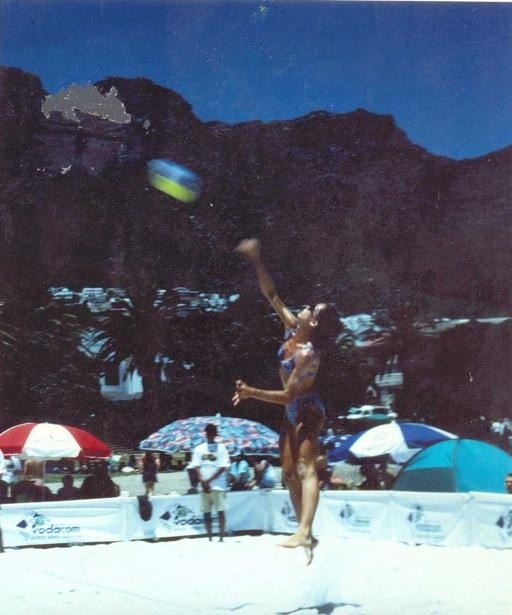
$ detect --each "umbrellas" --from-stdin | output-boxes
[138,413,281,458]
[0,422,113,501]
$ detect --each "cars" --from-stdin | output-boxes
[49,285,200,307]
[338,404,400,427]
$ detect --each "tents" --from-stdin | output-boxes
[390,438,512,493]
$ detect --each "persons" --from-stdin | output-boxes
[505,473,512,494]
[231,238,343,568]
[1,451,396,505]
[319,420,459,463]
[191,424,231,542]
